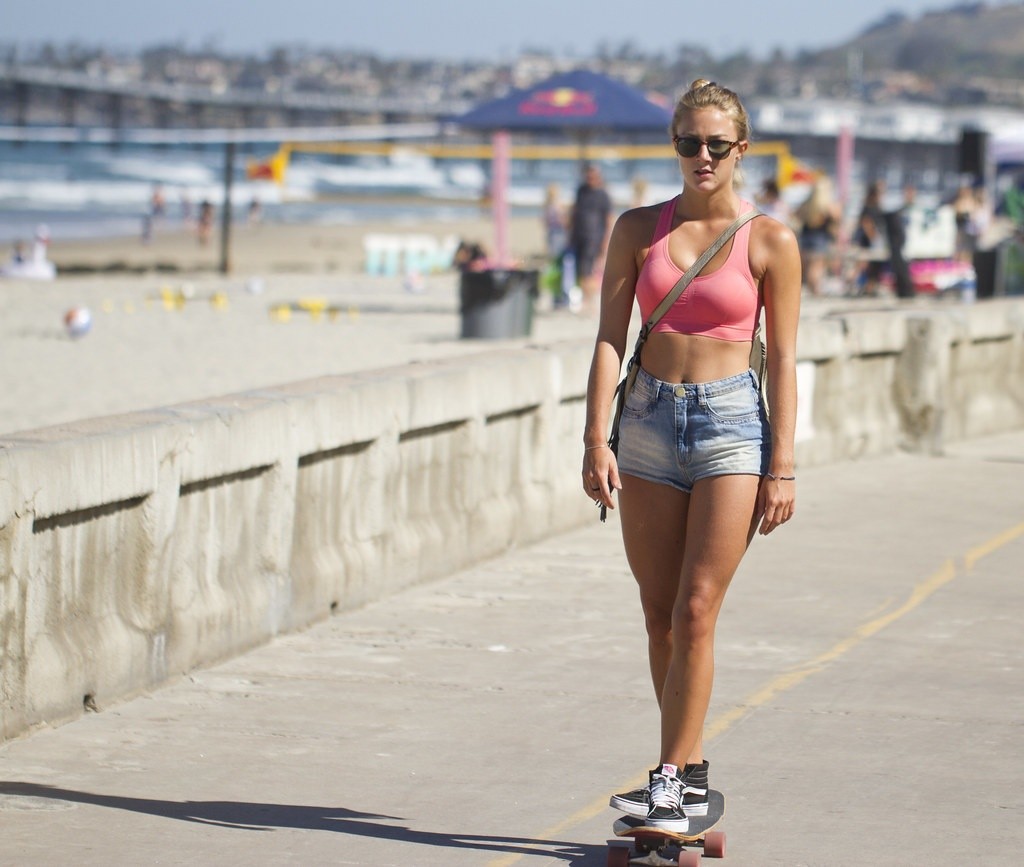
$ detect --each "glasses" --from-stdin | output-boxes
[672,128,737,160]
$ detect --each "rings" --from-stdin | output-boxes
[591,488,600,491]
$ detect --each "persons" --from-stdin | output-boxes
[134,179,260,251]
[579,76,802,834]
[532,183,583,315]
[565,159,614,317]
[750,160,1024,298]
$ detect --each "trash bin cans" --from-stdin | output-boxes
[459,256,539,339]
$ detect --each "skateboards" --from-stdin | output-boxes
[606,788,727,867]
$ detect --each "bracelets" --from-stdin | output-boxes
[766,471,796,481]
[584,444,607,450]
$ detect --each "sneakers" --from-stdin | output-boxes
[609,759,710,835]
